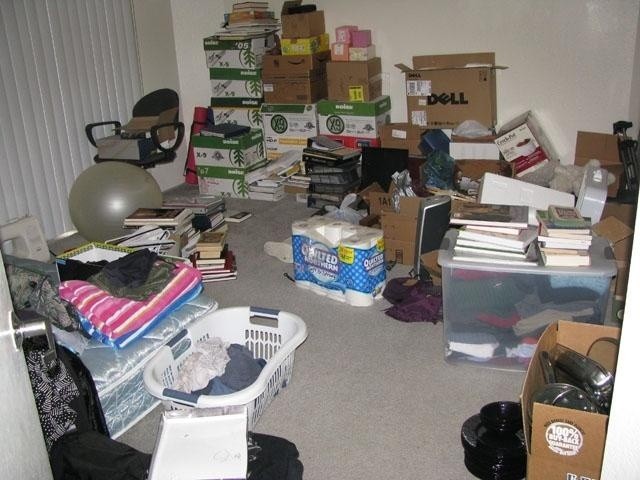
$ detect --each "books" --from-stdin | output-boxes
[448,198,595,268]
[245,150,311,201]
[212,1,282,40]
[122,194,252,283]
[199,122,251,138]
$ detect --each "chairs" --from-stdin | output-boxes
[81,86,186,168]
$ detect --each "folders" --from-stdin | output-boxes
[307,135,345,152]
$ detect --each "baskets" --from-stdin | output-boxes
[142,306,307,432]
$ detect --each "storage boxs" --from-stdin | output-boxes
[188,2,640,373]
[517,317,626,480]
[55,242,134,266]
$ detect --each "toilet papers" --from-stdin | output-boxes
[291,215,387,307]
